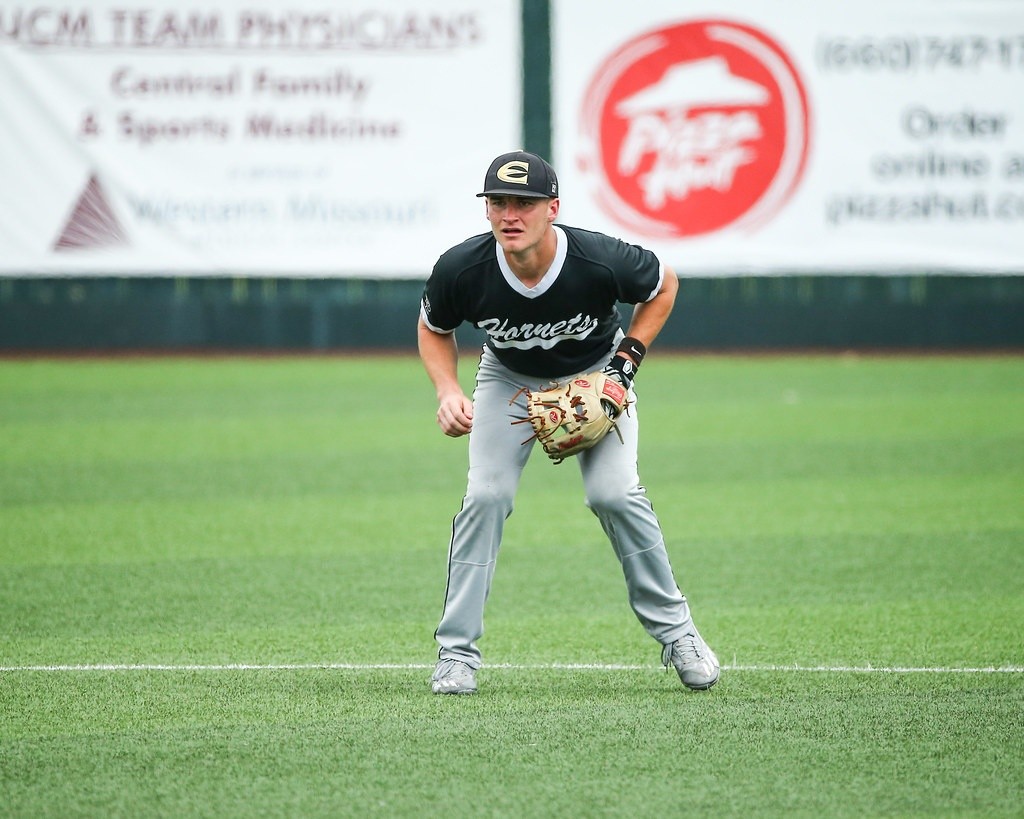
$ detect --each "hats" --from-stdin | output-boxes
[475,152,558,199]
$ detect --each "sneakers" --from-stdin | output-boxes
[431,657,477,694]
[660,625,721,690]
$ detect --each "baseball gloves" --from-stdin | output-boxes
[525,371,629,460]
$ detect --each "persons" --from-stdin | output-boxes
[418,152,719,691]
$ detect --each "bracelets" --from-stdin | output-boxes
[616,337,646,366]
